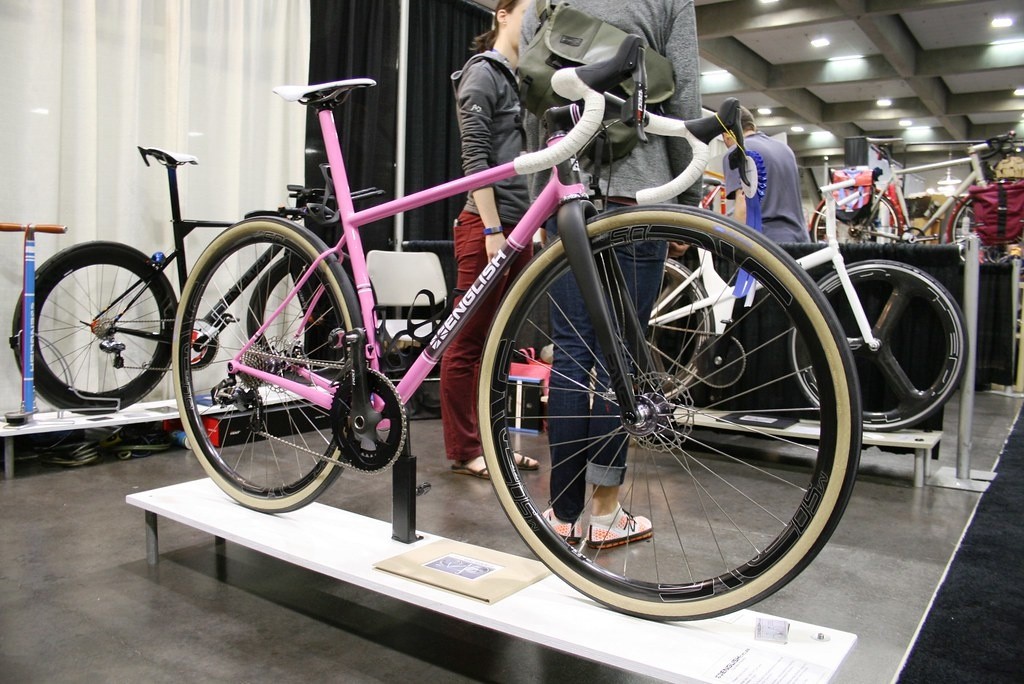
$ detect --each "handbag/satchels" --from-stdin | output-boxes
[508,347,552,433]
[968,177,1024,245]
[834,166,873,222]
[517,1,675,166]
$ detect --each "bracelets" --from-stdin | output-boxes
[483,225,504,235]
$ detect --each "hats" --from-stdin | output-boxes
[741,104,755,129]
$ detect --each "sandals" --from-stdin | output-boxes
[450,455,490,478]
[513,453,541,470]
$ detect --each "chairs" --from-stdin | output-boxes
[362,250,448,408]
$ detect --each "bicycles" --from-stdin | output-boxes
[813,131,1024,262]
[172,34,862,622]
[649,177,970,434]
[7,146,387,419]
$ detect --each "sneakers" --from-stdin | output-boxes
[542,508,582,544]
[585,507,652,548]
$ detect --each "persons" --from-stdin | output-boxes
[519,0,705,550]
[442,0,542,480]
[722,103,813,245]
